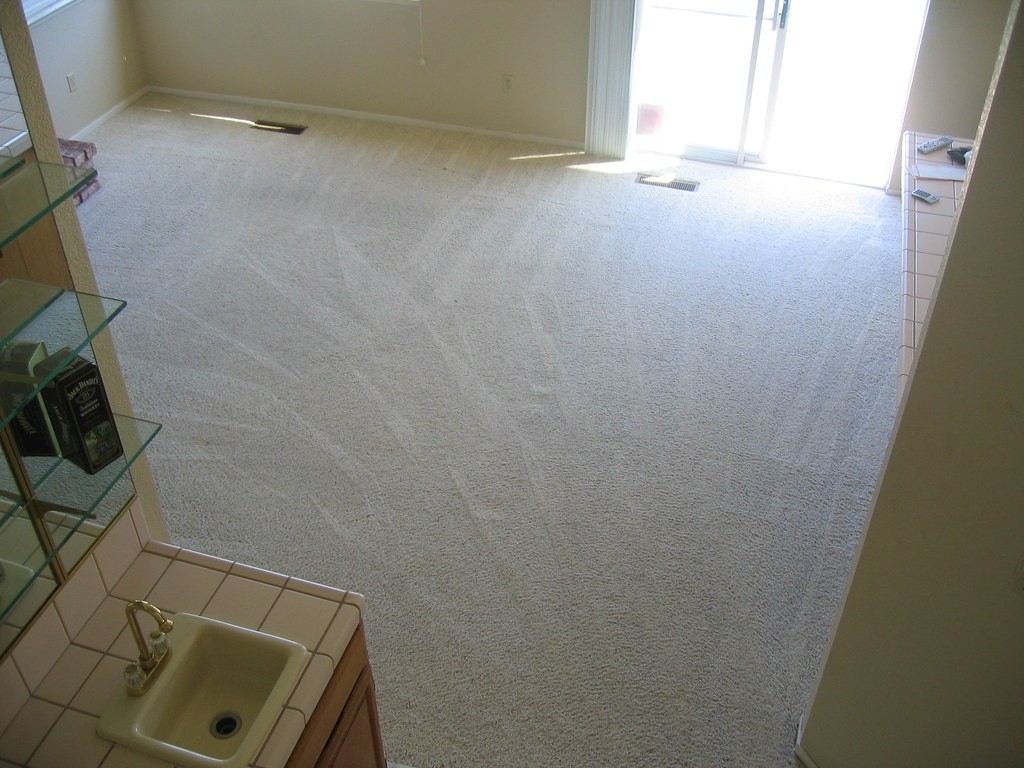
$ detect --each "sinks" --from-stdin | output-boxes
[134,623,308,768]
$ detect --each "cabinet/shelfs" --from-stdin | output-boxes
[0,0,389,768]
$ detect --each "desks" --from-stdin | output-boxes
[900,128,976,403]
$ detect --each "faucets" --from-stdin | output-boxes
[119,599,174,697]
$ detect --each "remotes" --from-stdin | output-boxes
[911,189,940,204]
[918,136,953,155]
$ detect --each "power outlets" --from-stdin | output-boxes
[66,73,78,93]
